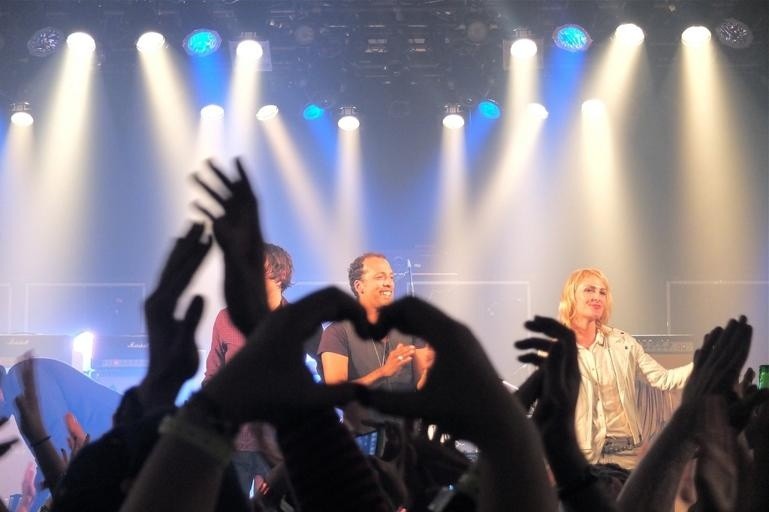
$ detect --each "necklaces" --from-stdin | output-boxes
[372,339,386,367]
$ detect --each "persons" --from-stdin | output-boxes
[201,240,324,500]
[1,154,768,512]
[315,252,435,434]
[557,267,694,473]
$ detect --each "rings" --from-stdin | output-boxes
[397,356,402,361]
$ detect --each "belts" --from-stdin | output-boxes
[601,436,642,454]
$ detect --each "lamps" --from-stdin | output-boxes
[10,19,753,132]
